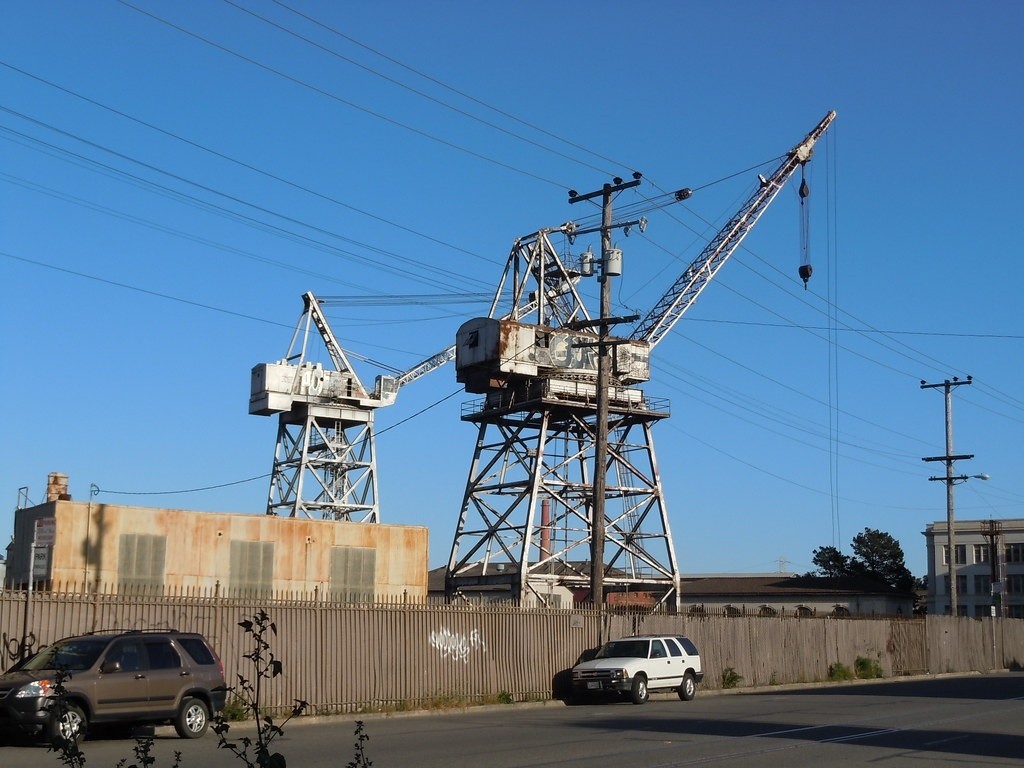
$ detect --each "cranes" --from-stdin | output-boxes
[445,110,838,613]
[248,271,581,524]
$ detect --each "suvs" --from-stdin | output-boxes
[0,628,226,748]
[571,633,704,704]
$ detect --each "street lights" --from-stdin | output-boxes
[946,473,990,616]
